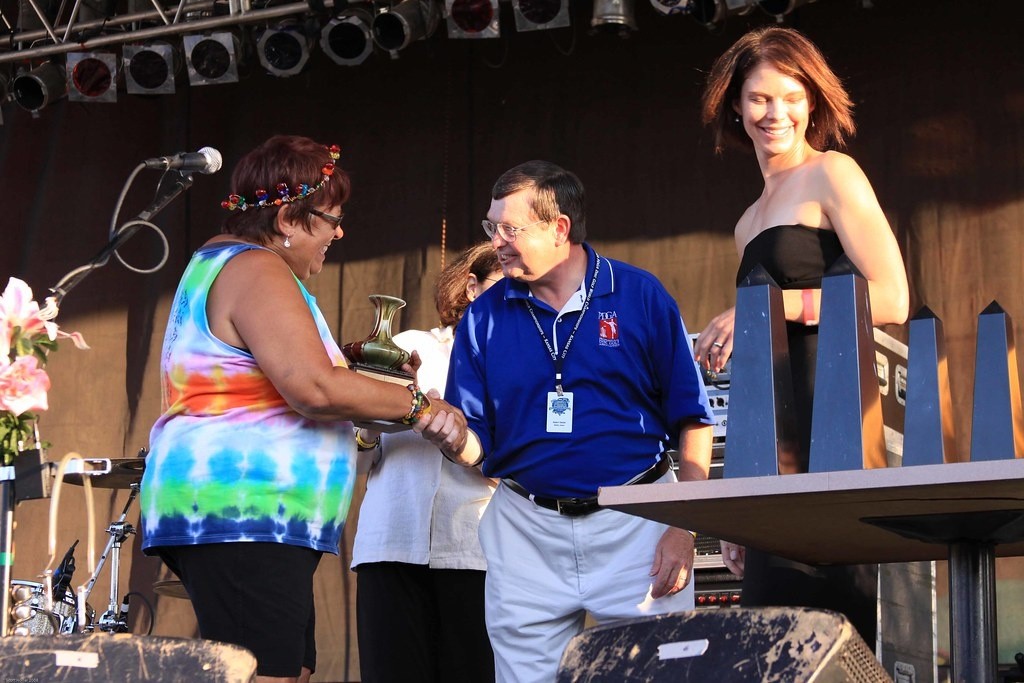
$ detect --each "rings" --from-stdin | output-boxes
[713,342,723,348]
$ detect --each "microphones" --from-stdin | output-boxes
[118,595,130,633]
[140,145,223,175]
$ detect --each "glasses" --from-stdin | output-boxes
[301,205,345,231]
[483,218,545,244]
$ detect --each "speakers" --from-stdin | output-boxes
[556,602,899,683]
[0,634,258,683]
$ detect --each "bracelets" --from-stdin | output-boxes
[401,383,432,424]
[355,428,381,451]
[802,290,814,326]
[689,530,697,538]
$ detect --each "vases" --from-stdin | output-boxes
[341,295,411,370]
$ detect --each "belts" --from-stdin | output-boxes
[502,452,671,516]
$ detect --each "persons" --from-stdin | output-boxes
[412,160,716,683]
[356,238,511,683]
[139,134,469,683]
[693,27,913,650]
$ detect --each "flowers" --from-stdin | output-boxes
[0,276,94,465]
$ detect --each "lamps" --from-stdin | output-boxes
[0,0,806,126]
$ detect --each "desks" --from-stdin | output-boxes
[597,455,1024,683]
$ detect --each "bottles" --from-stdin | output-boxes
[341,295,410,372]
